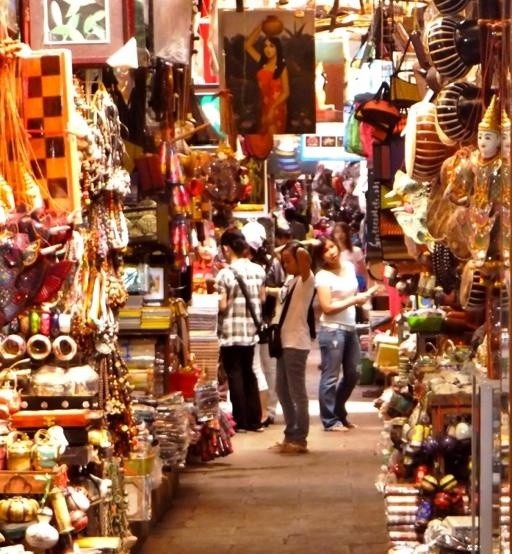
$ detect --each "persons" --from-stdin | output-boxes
[245,23,289,134]
[440,95,500,259]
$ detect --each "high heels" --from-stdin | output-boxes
[237,414,274,432]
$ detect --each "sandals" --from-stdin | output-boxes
[324,422,358,432]
[268,442,307,455]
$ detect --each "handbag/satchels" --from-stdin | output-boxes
[256,323,283,358]
[344,74,422,181]
